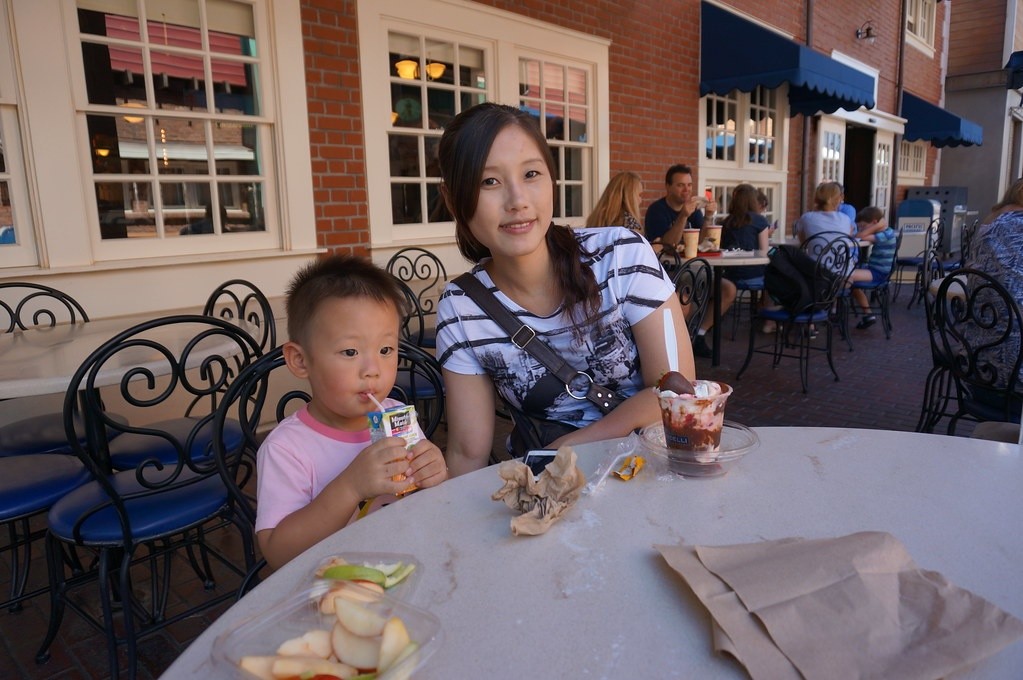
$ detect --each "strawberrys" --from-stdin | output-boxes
[656,370,696,394]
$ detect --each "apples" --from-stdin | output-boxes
[237,565,419,680]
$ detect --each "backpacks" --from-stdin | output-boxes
[764,244,836,314]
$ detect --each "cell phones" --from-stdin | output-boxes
[521,448,559,483]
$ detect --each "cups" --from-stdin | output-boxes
[705,225,723,252]
[683,229,700,259]
[653,380,734,470]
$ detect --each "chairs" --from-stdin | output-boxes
[0,218,1023,680]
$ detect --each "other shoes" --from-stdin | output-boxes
[856,316,876,329]
[804,329,816,339]
[763,323,783,334]
[692,335,713,358]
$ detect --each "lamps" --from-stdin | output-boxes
[394,55,445,82]
[94,145,113,157]
[856,20,877,44]
[122,104,147,123]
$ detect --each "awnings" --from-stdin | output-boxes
[900,90,983,148]
[699,0,875,118]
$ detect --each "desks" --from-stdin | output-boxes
[0,317,258,613]
[661,249,770,367]
[159,426,1023,679]
[769,237,870,248]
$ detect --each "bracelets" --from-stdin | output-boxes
[704,216,712,221]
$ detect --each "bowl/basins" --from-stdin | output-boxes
[638,418,761,478]
[212,580,444,680]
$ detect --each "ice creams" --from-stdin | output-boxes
[655,377,725,471]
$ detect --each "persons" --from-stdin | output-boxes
[832,182,860,261]
[435,102,696,477]
[584,172,663,255]
[645,163,737,358]
[962,176,1023,423]
[797,181,854,338]
[179,202,229,236]
[255,256,447,569]
[832,207,896,329]
[756,191,779,238]
[721,184,782,333]
